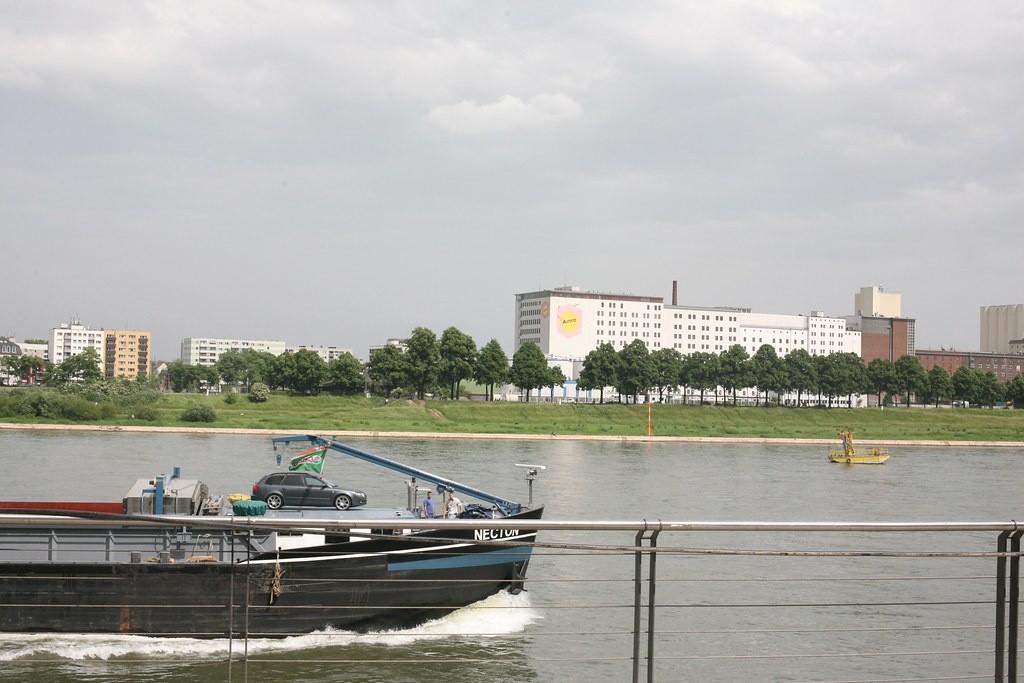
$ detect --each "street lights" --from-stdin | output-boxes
[885,326,891,364]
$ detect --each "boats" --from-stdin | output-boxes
[1,433,547,637]
[827,429,891,465]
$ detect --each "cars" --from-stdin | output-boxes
[249,470,367,511]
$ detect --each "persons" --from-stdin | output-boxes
[385,399,389,404]
[423,491,435,520]
[444,492,462,520]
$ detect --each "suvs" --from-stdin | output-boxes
[561,398,575,405]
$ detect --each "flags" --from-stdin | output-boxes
[288,443,329,476]
